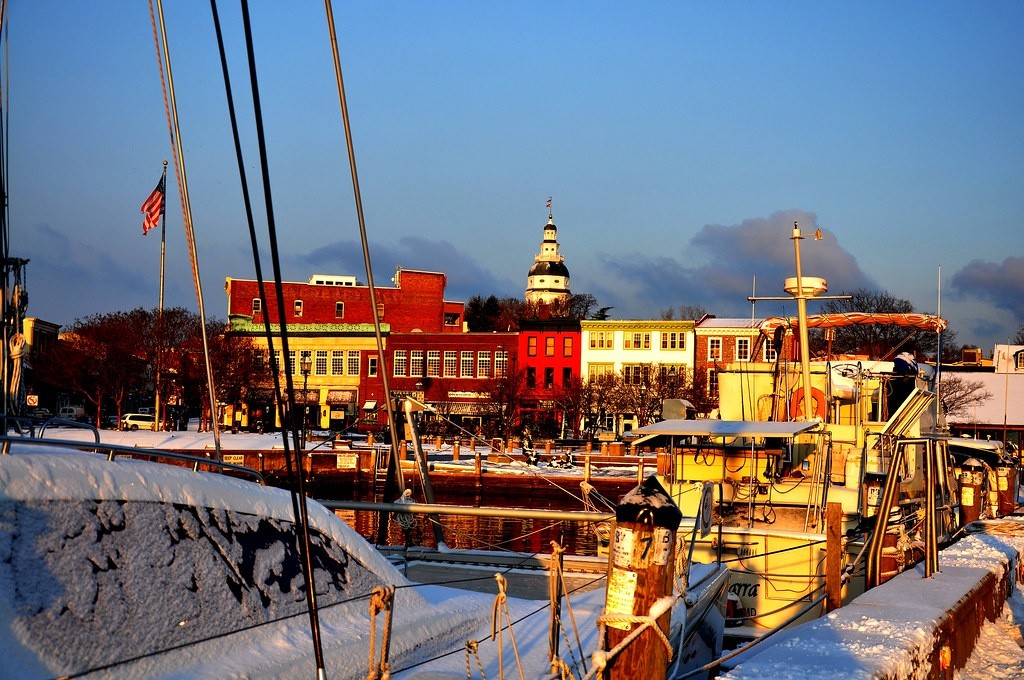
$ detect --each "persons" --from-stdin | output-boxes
[522,428,540,467]
[550,453,572,467]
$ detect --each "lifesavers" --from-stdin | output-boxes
[788,385,831,424]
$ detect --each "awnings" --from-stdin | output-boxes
[280,389,320,405]
[244,388,275,403]
[326,390,357,405]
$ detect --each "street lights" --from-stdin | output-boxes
[300,352,312,450]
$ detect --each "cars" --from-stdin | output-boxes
[139,406,155,415]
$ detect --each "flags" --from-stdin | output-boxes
[140,167,166,236]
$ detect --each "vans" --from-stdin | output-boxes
[57,404,85,421]
[121,412,170,432]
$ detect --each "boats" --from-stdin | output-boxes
[601,221,963,680]
